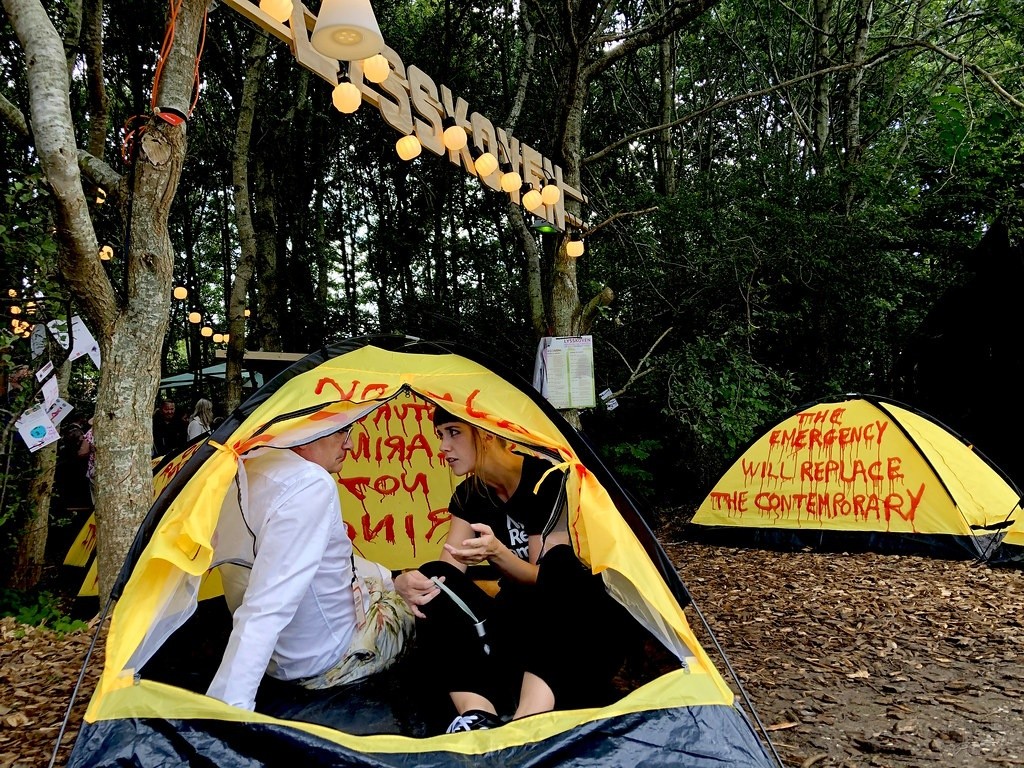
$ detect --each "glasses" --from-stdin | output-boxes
[341,428,352,443]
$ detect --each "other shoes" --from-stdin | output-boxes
[445,709,507,734]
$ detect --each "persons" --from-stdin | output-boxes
[187,396,215,441]
[153,399,187,457]
[394,404,647,735]
[202,423,414,716]
[78,417,96,506]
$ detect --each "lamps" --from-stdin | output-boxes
[201,326,213,337]
[472,145,499,177]
[566,232,584,257]
[245,307,251,317]
[541,178,560,205]
[213,332,224,343]
[224,334,230,342]
[520,183,543,212]
[364,55,390,83]
[256,0,294,24]
[332,70,362,114]
[5,184,115,341]
[442,116,467,151]
[174,284,187,300]
[188,311,201,323]
[501,162,523,193]
[310,0,385,63]
[396,130,422,162]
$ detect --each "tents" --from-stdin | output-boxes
[49,328,784,768]
[676,393,1023,569]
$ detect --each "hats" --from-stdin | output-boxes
[432,404,463,426]
